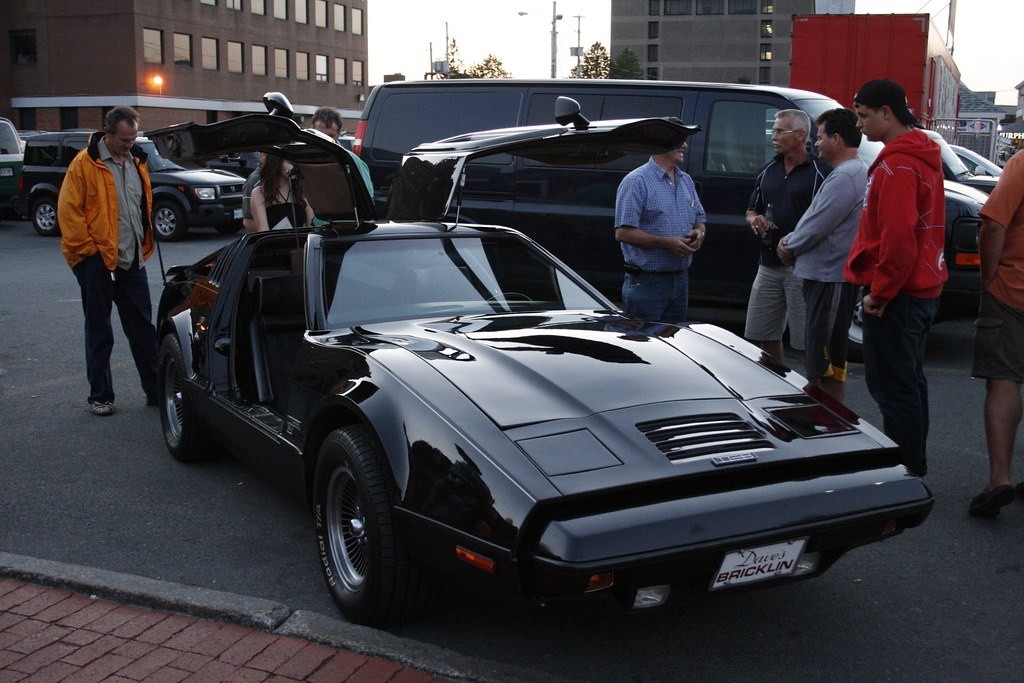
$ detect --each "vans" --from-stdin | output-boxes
[350,79,991,365]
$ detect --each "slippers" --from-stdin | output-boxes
[1015,481,1024,498]
[969,483,1015,514]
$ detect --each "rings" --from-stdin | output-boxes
[755,225,759,228]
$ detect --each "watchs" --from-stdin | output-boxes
[701,229,705,239]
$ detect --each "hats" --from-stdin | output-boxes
[854,78,917,124]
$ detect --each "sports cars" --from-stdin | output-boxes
[143,92,934,632]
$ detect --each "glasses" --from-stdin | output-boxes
[771,128,804,135]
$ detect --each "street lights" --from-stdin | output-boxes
[518,1,563,78]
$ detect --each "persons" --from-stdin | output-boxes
[57,105,158,415]
[745,78,947,479]
[615,116,707,323]
[968,148,1024,519]
[242,107,377,232]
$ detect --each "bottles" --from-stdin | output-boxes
[762,203,774,246]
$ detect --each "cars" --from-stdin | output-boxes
[21,132,248,242]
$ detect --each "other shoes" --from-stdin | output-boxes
[146,399,160,407]
[90,400,114,416]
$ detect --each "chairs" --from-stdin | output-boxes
[401,264,473,301]
[248,275,304,402]
